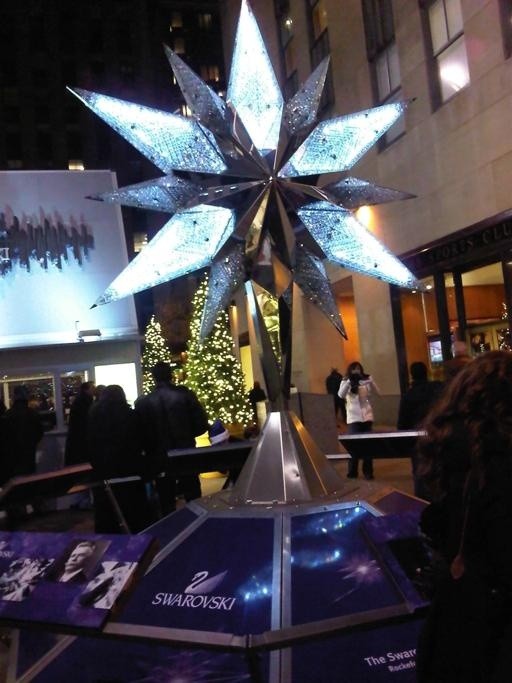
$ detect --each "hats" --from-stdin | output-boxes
[209,420,230,445]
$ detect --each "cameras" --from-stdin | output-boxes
[350,374,363,393]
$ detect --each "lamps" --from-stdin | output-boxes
[78,329,102,341]
[67,158,85,171]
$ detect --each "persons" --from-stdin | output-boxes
[45,539,96,586]
[81,564,129,609]
[338,362,378,480]
[208,420,253,487]
[397,362,441,498]
[250,381,267,420]
[327,367,346,419]
[413,350,509,683]
[133,364,208,501]
[0,375,158,534]
[444,341,470,388]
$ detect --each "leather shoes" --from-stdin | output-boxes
[365,471,373,479]
[348,471,357,479]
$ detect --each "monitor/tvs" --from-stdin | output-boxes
[429,340,455,362]
